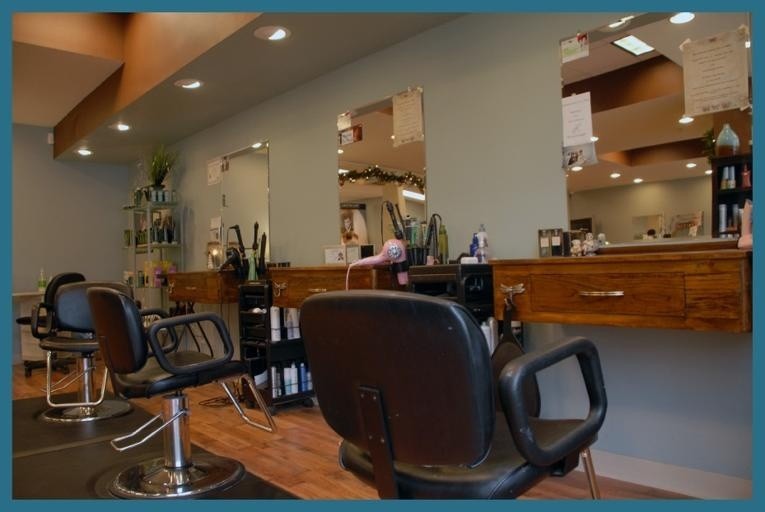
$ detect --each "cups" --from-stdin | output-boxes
[138,260,176,287]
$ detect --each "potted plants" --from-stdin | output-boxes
[143,143,179,205]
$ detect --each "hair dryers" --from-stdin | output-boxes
[353,239,409,286]
[217,246,244,279]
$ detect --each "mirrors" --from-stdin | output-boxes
[220,139,270,269]
[559,12,752,248]
[337,86,427,259]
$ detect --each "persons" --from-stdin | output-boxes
[343,214,353,233]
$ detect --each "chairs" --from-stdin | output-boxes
[83,283,278,500]
[295,287,608,500]
[13,271,138,426]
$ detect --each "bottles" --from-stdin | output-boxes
[137,214,177,244]
[37,267,45,290]
[719,163,752,190]
[133,187,177,206]
[271,362,311,400]
[716,123,738,155]
[538,228,563,256]
[439,225,449,263]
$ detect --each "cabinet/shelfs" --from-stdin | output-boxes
[711,153,752,238]
[119,202,188,352]
[166,250,750,334]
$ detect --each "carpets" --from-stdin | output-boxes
[12,386,304,498]
